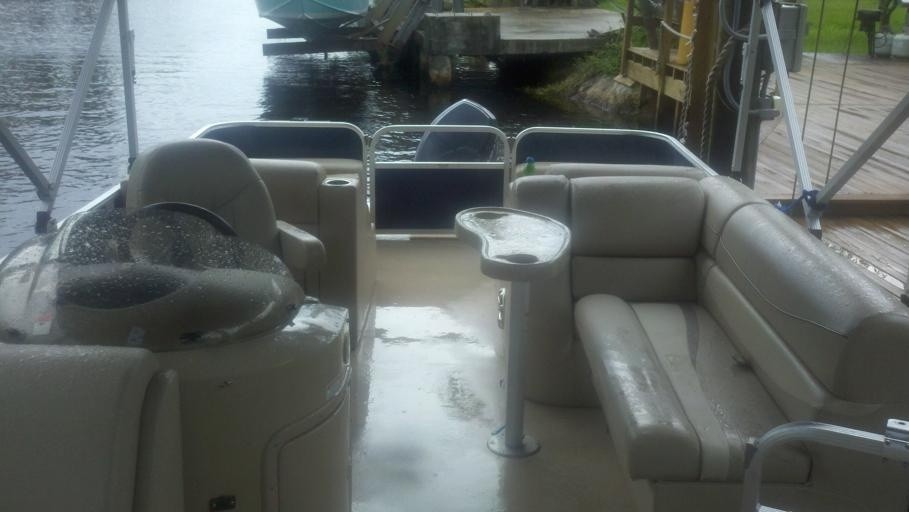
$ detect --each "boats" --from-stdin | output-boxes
[0,0,909,512]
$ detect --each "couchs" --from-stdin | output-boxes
[505,158,909,511]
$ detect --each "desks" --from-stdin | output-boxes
[452,204,572,458]
